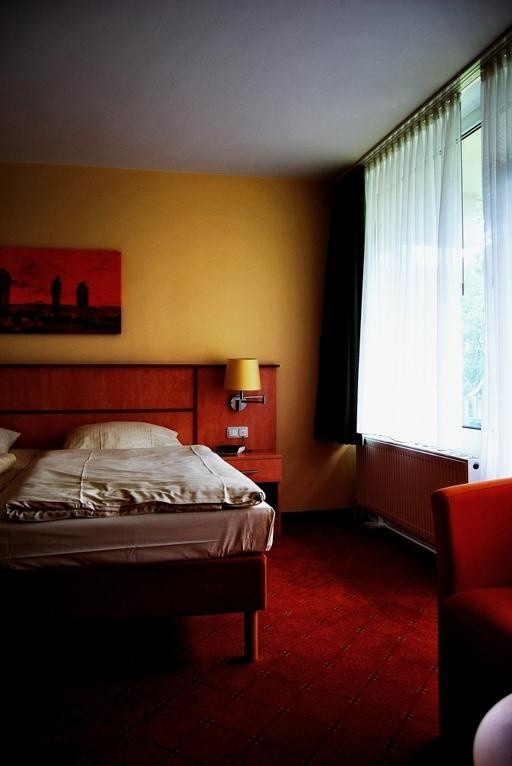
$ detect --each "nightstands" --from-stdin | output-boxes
[219,450,283,538]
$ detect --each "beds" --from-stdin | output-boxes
[2,444,277,662]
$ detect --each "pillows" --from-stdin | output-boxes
[62,420,183,448]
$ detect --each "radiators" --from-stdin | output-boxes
[355,436,481,550]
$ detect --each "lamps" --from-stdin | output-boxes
[223,358,266,411]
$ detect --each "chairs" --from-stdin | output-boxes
[429,480,510,738]
